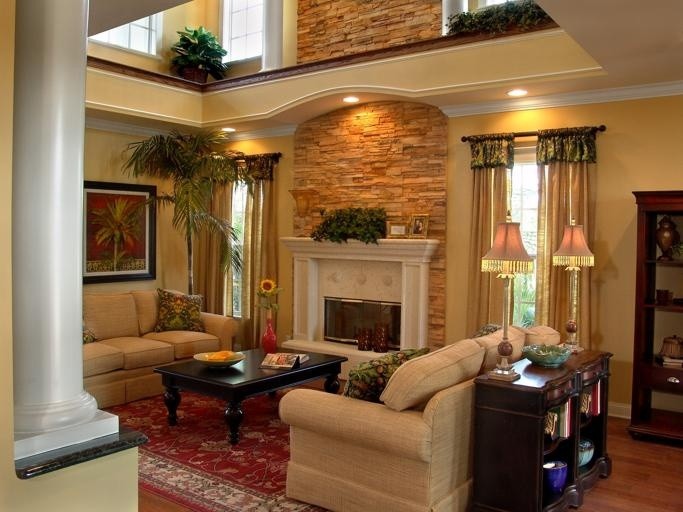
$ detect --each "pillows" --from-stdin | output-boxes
[343,346,432,399]
[82,322,95,345]
[153,288,205,333]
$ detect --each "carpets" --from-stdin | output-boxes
[103,370,349,510]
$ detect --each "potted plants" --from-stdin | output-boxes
[168,27,227,86]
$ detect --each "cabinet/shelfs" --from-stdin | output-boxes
[471,343,612,509]
[626,189,683,446]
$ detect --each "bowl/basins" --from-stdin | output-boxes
[521,344,571,368]
[543,461,568,492]
[579,440,595,468]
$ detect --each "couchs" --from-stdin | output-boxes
[82,289,239,407]
[278,325,560,510]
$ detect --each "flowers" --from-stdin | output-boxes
[254,279,281,321]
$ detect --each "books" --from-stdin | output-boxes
[662,354,683,368]
[556,398,571,439]
[591,379,600,416]
[258,353,310,370]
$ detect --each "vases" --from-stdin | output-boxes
[261,319,279,356]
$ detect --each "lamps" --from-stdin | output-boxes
[479,216,533,381]
[552,222,595,346]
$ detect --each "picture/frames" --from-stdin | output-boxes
[83,180,158,284]
[404,213,429,238]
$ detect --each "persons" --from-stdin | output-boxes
[414,219,422,234]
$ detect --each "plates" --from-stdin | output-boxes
[192,352,246,368]
